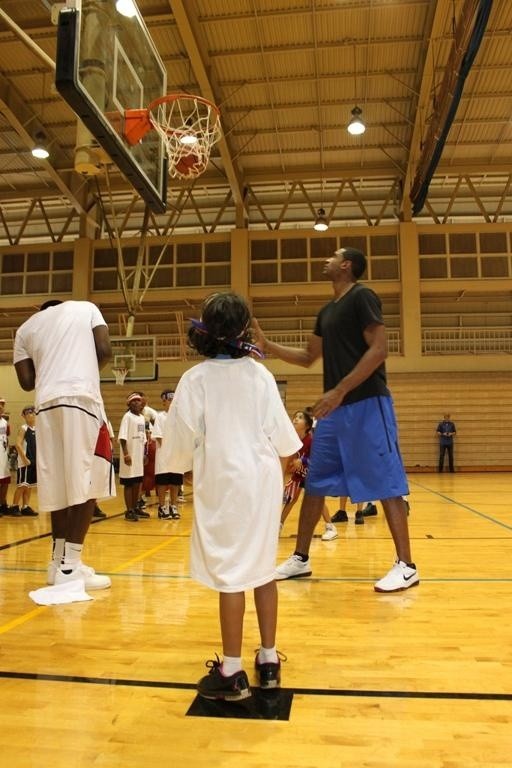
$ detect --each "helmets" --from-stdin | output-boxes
[22,405,35,415]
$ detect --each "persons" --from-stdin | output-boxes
[329,497,365,525]
[305,406,317,435]
[160,292,304,702]
[363,494,408,518]
[248,247,419,593]
[278,411,338,541]
[436,413,456,473]
[0,388,183,522]
[13,300,110,591]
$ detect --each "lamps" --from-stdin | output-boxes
[313,130,328,232]
[32,70,50,158]
[346,44,366,134]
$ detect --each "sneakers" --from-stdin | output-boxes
[254,649,287,691]
[362,503,377,516]
[169,504,181,519]
[94,506,106,517]
[196,652,252,703]
[142,494,147,501]
[321,526,338,541]
[275,554,312,581]
[158,504,172,520]
[137,499,147,509]
[10,505,22,516]
[21,506,39,516]
[134,507,149,518]
[374,561,419,593]
[0,504,10,514]
[331,510,348,522]
[54,567,111,591]
[47,563,96,586]
[355,511,363,524]
[176,495,187,502]
[125,510,139,521]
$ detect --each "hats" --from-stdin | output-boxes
[0,399,8,403]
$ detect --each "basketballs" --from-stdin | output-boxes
[172,143,208,175]
